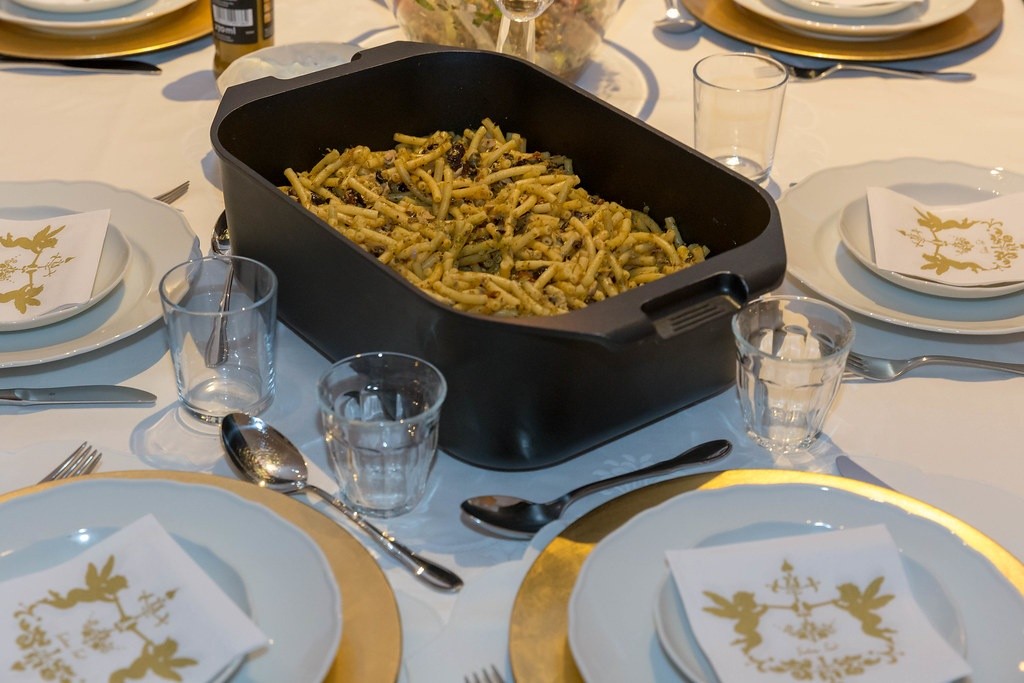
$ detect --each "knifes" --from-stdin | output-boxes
[0,57,162,76]
[0,385,158,407]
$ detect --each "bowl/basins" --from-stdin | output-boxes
[13,0,138,13]
[779,0,917,17]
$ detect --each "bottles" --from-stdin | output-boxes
[210,0,274,79]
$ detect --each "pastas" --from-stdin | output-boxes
[274,117,708,318]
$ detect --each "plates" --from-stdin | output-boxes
[774,156,1024,335]
[682,0,1005,60]
[0,479,341,683]
[840,181,1024,298]
[0,470,403,683]
[656,521,966,683]
[216,42,366,96]
[0,207,133,331]
[567,483,1024,683]
[732,0,977,42]
[0,0,213,58]
[0,526,249,683]
[0,179,202,369]
[0,0,195,33]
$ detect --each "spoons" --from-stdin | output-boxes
[459,438,733,540]
[653,1,700,33]
[222,413,463,592]
[201,208,234,368]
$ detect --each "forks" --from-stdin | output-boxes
[37,441,102,484]
[152,180,190,204]
[754,45,975,83]
[463,664,506,683]
[813,331,1024,382]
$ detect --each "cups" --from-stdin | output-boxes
[317,352,447,517]
[159,255,278,423]
[694,51,789,185]
[730,295,853,454]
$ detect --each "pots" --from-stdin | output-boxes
[211,41,788,473]
